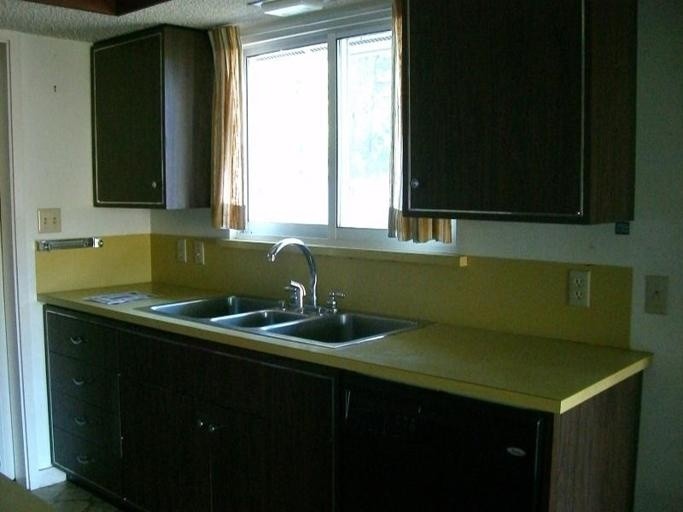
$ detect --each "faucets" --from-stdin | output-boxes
[265,237,319,307]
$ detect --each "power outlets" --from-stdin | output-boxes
[567,269,592,308]
[645,273,669,316]
[36,207,62,233]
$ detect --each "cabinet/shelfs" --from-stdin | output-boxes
[120,322,333,511]
[42,303,121,509]
[393,1,638,225]
[89,23,215,210]
[332,372,639,512]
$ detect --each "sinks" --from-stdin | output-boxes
[259,312,419,348]
[148,295,276,319]
[210,310,309,330]
